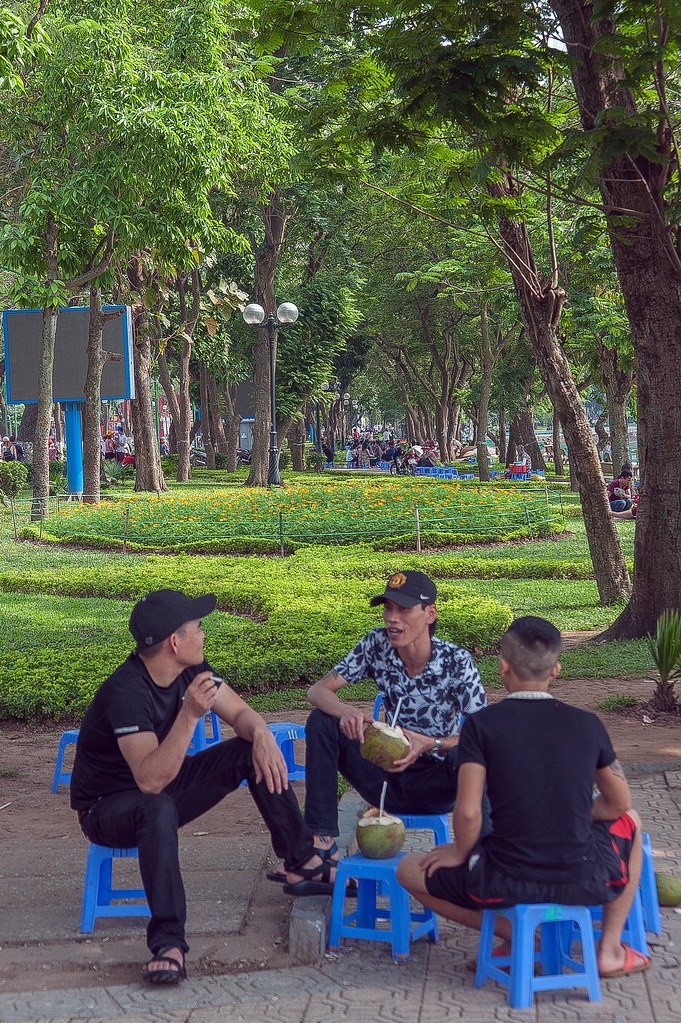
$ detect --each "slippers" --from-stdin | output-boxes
[472,946,540,972]
[600,944,654,978]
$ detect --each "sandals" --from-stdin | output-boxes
[267,842,339,881]
[142,939,190,985]
[281,853,358,897]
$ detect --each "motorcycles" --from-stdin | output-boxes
[390,446,417,476]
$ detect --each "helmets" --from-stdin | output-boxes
[3,436,10,441]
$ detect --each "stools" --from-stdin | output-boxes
[555,883,648,960]
[476,901,604,1007]
[48,728,78,795]
[80,842,152,937]
[240,719,308,789]
[319,439,547,484]
[637,832,664,936]
[375,816,452,898]
[326,852,440,958]
[184,706,220,761]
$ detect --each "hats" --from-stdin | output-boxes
[515,444,524,449]
[371,570,438,610]
[118,427,123,431]
[106,430,114,436]
[129,588,217,645]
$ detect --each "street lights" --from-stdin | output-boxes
[243,302,298,488]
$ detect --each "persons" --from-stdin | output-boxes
[100,425,127,464]
[0,433,23,462]
[542,446,610,465]
[159,436,169,456]
[606,470,633,510]
[267,569,489,884]
[609,502,637,520]
[394,616,648,983]
[319,427,423,468]
[69,589,361,989]
[610,460,635,495]
[49,435,59,461]
[515,444,532,473]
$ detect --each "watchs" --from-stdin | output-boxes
[429,737,442,755]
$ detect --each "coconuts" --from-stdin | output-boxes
[359,721,411,771]
[356,808,406,859]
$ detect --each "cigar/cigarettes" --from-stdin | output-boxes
[210,674,224,683]
